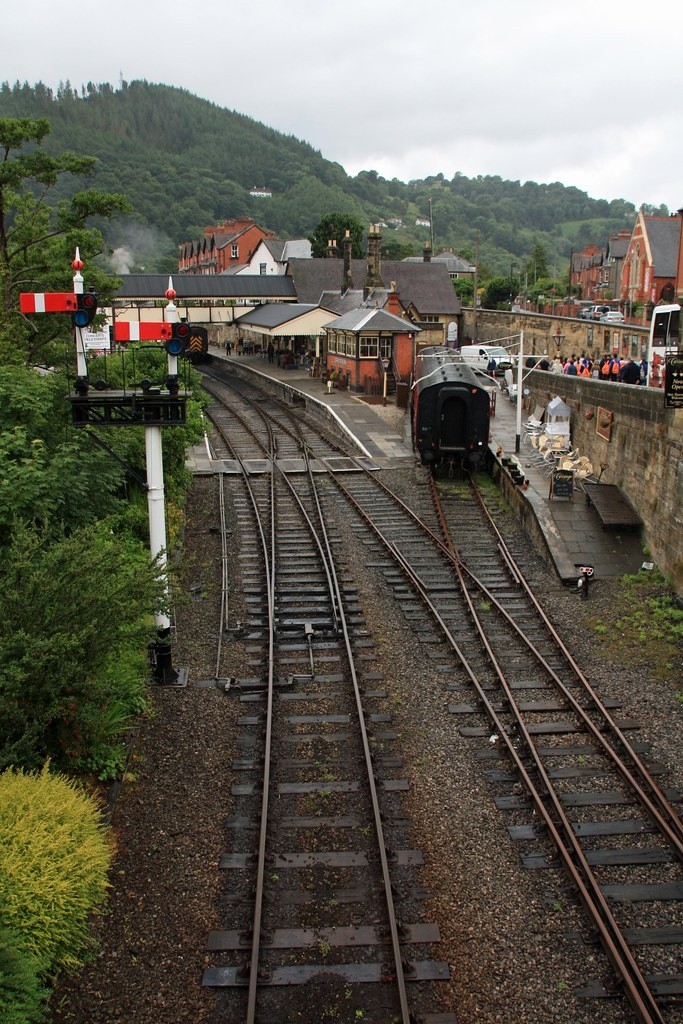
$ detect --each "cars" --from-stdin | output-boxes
[590,306,610,321]
[541,295,563,307]
[564,297,574,304]
[599,312,625,324]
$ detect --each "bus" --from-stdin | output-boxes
[647,304,681,388]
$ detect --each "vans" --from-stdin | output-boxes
[461,346,515,375]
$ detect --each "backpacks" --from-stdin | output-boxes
[602,362,610,374]
[613,359,619,374]
[582,365,589,378]
[593,369,598,377]
[620,362,625,367]
[590,361,595,365]
[576,365,581,375]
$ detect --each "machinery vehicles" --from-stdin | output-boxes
[429,199,434,257]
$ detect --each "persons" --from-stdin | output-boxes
[222,335,308,366]
[522,349,648,385]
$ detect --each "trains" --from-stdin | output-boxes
[181,327,208,365]
[410,347,490,480]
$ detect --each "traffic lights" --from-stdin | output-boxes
[73,309,89,326]
[82,296,95,309]
[176,324,190,337]
[165,338,183,356]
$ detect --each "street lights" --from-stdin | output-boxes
[552,335,566,358]
[629,249,634,325]
[517,271,527,310]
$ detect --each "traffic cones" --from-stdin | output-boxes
[492,371,496,380]
